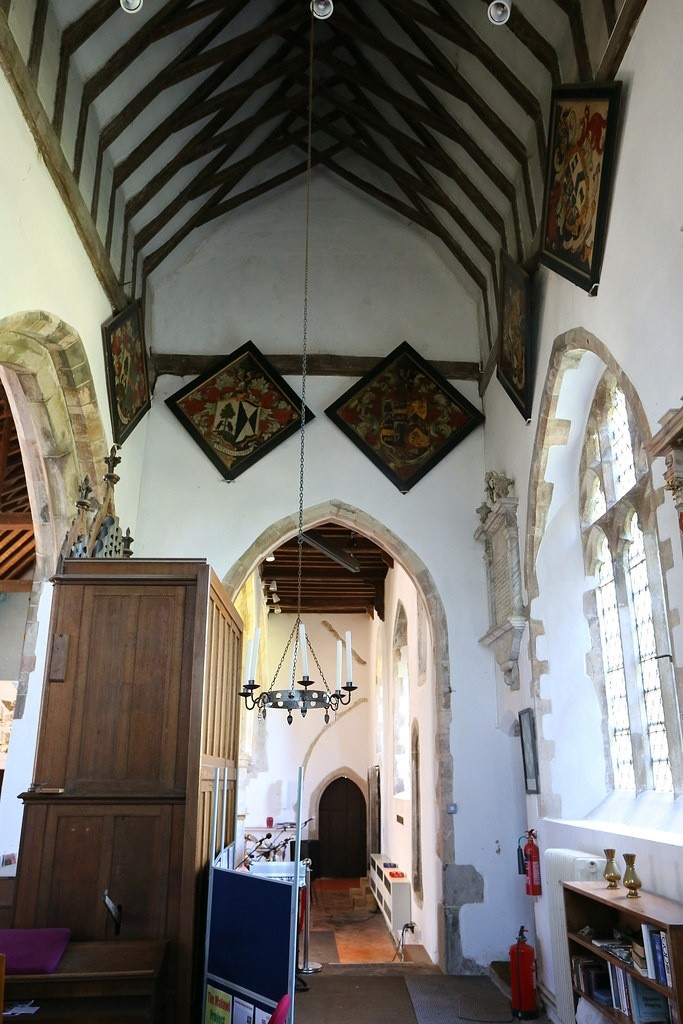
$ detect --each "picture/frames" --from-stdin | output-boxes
[496,247,534,424]
[324,341,486,493]
[518,707,540,795]
[537,79,624,296]
[163,339,315,484]
[103,297,151,450]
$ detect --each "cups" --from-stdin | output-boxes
[266,817,272,827]
[603,849,642,898]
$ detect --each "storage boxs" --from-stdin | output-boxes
[350,878,377,912]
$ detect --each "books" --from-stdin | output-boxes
[572,921,672,1024]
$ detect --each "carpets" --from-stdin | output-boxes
[403,973,519,1024]
[457,992,514,1022]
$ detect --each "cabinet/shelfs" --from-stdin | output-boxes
[11,557,238,1020]
[562,880,683,1024]
[369,854,410,929]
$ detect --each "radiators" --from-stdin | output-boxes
[543,847,608,1024]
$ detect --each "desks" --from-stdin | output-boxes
[0,939,175,1024]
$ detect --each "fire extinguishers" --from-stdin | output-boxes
[509,925,537,1019]
[524,829,542,896]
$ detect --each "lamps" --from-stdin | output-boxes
[309,0,334,20]
[120,0,143,14]
[241,0,358,725]
[487,0,513,26]
